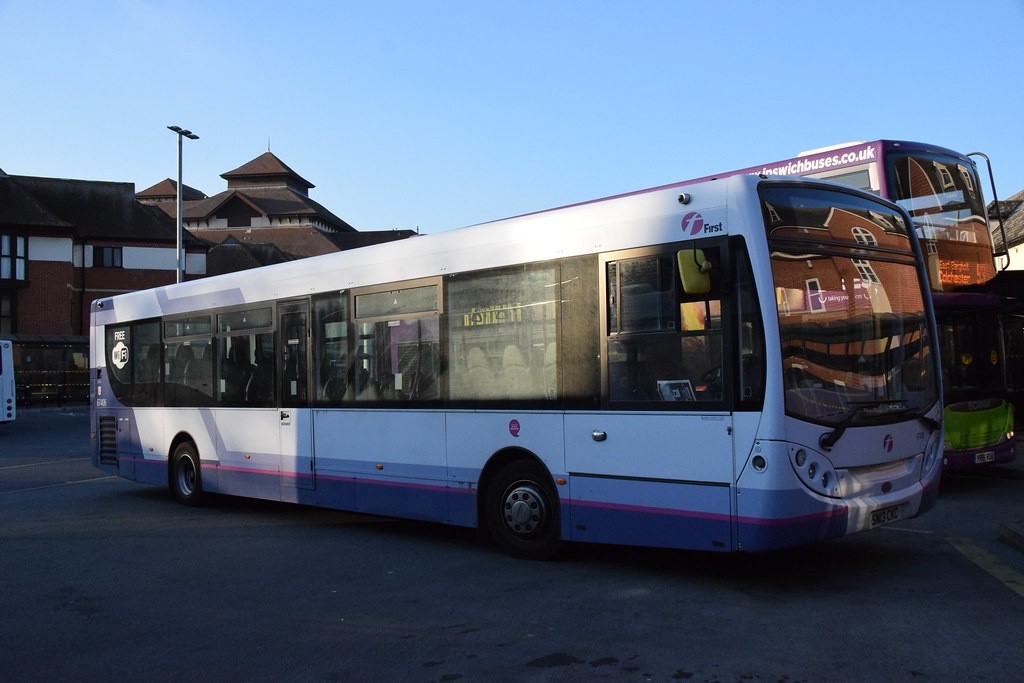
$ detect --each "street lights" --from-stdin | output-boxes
[166,125,200,284]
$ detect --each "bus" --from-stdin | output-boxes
[90,173,944,560]
[728,139,1019,472]
[0,340,16,422]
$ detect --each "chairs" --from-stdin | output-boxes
[136,342,558,400]
